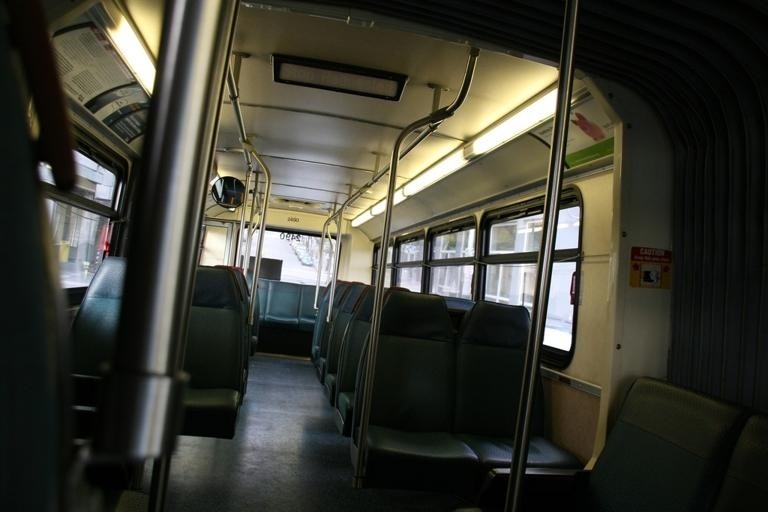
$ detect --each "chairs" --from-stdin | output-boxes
[249,278,327,348]
[64,256,263,456]
